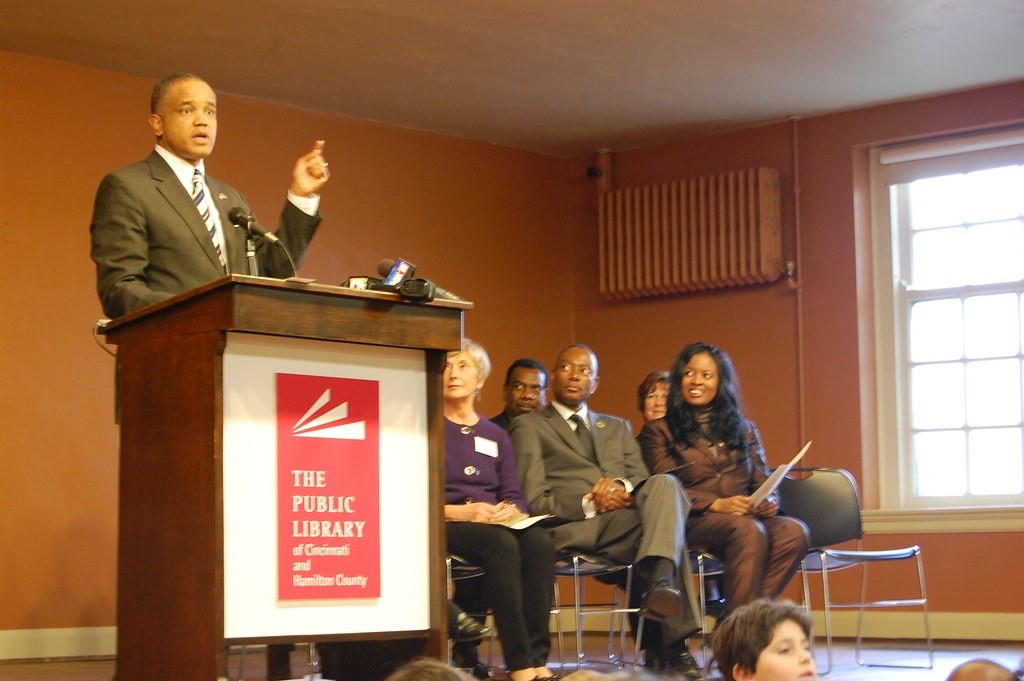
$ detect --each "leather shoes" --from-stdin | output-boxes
[663,647,701,678]
[639,579,680,622]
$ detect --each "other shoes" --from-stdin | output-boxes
[453,648,494,678]
[452,617,490,641]
[508,666,537,681]
[534,665,560,681]
[450,554,486,579]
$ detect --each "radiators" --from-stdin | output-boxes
[598,167,799,301]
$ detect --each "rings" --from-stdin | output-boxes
[610,488,615,491]
[324,163,328,167]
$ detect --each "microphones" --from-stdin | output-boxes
[377,258,465,303]
[340,275,435,303]
[228,207,285,248]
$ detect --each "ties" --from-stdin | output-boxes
[192,169,226,274]
[570,414,595,461]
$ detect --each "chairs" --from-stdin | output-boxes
[307,466,935,681]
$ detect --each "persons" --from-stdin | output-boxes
[385,658,478,681]
[508,344,704,681]
[637,370,719,612]
[639,342,811,616]
[488,358,550,430]
[88,72,331,318]
[712,599,818,681]
[443,338,559,681]
